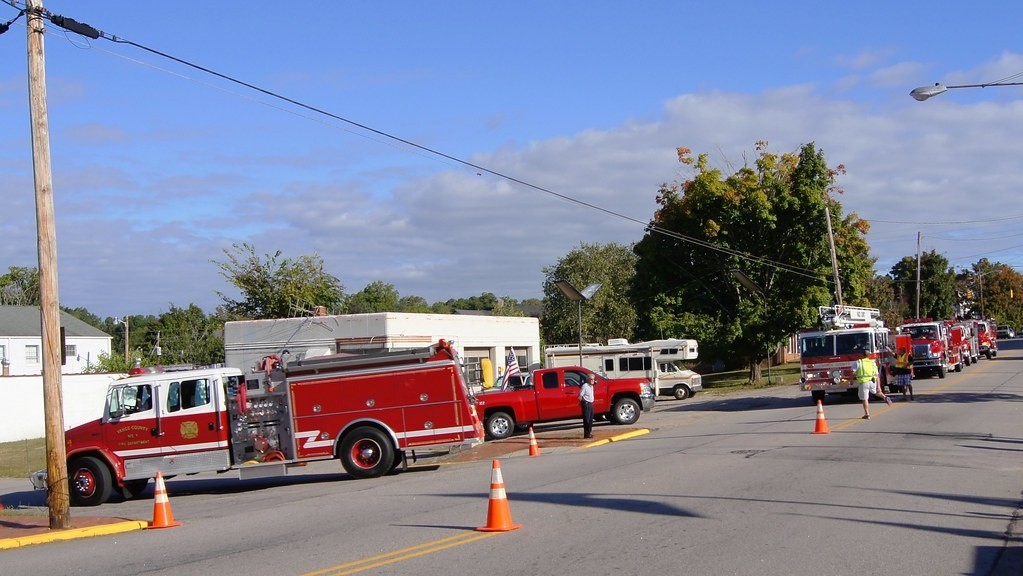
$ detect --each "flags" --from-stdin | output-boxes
[501,345,521,392]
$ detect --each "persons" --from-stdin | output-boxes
[853,350,893,420]
[577,373,595,438]
[885,345,916,401]
[133,385,152,413]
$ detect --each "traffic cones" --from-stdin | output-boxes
[475,459,522,533]
[809,400,832,434]
[528,427,541,457]
[142,468,183,530]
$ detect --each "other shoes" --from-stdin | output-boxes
[585,434,594,438]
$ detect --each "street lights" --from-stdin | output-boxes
[553,279,604,367]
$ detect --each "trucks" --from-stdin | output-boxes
[544,338,703,401]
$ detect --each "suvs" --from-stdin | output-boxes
[996,325,1014,339]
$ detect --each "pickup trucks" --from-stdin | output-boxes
[472,365,656,440]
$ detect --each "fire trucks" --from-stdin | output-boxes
[796,305,914,401]
[29,339,485,507]
[894,317,998,379]
